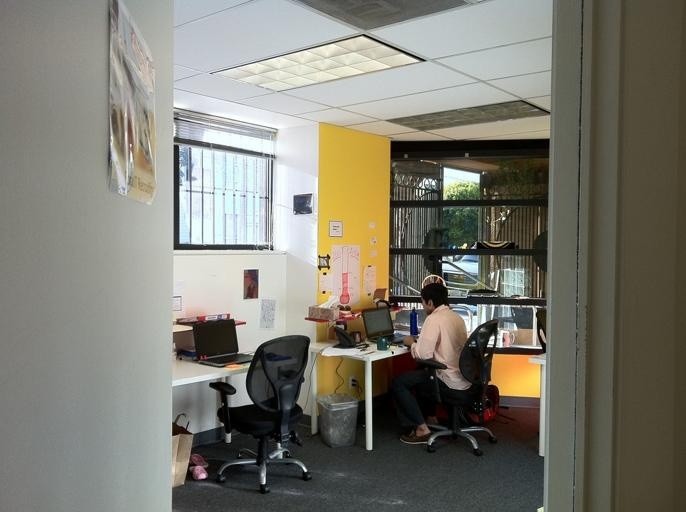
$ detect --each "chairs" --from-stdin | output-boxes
[209,334,313,493]
[417,319,501,457]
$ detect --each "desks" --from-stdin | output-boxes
[172,352,253,444]
[310,340,413,452]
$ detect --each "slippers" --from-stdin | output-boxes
[190,454,208,468]
[189,465,208,480]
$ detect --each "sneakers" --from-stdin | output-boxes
[399,430,436,445]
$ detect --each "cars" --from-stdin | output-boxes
[441,247,479,284]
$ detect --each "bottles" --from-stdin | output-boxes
[409,308,419,337]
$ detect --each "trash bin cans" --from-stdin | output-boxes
[316,392,359,448]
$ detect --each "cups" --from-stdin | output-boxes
[501,330,515,346]
[377,337,390,351]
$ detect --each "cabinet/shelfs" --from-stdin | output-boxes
[390,157,549,350]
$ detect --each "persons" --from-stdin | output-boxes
[389,282,473,445]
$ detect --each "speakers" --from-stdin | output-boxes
[351,331,361,343]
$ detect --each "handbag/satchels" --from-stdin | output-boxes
[172,413,194,488]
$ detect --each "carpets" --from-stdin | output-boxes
[172,413,544,512]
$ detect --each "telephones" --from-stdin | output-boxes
[333,326,356,349]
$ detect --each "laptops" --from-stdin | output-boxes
[192,318,254,368]
[362,306,408,345]
